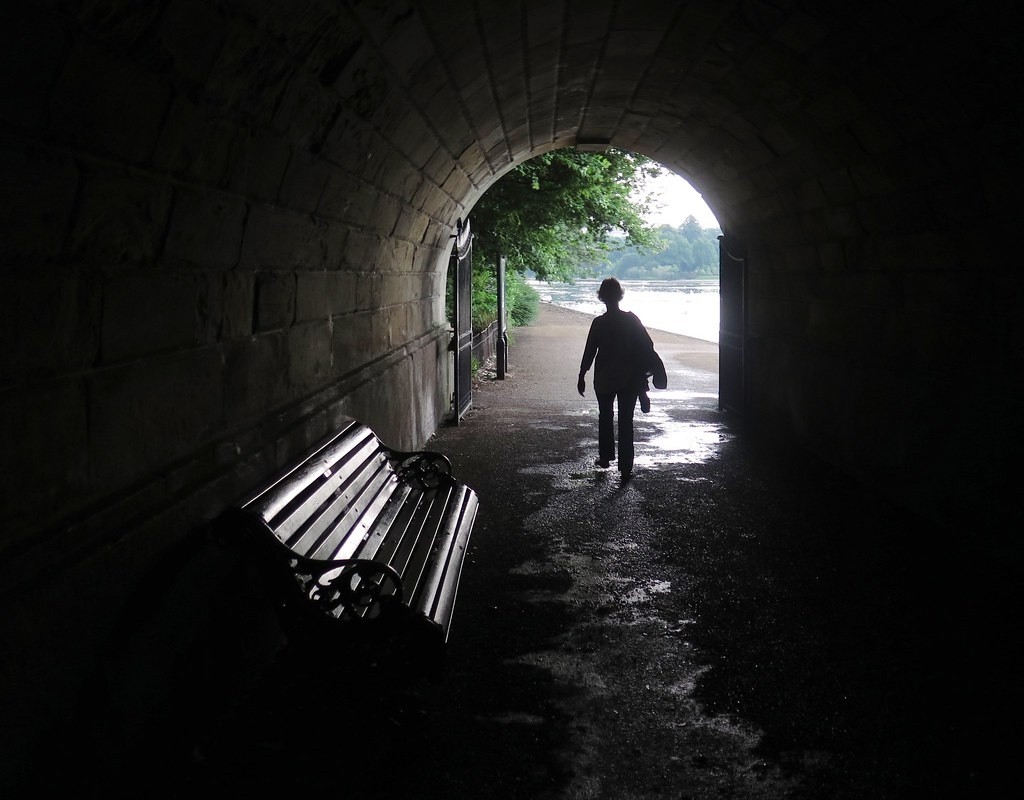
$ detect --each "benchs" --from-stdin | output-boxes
[231,419,479,734]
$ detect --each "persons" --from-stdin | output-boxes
[578,278,654,476]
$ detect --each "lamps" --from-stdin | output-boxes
[576,136,611,152]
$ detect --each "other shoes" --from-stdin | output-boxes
[620,470,631,476]
[594,458,610,469]
